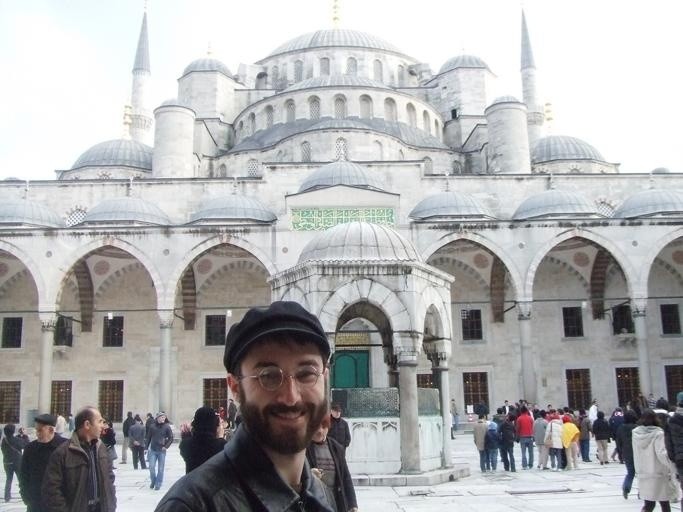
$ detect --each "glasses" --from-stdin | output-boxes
[236,365,324,391]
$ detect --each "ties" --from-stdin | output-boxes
[88,448,98,500]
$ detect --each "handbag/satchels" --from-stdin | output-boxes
[544,434,553,449]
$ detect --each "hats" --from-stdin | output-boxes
[222,300,332,375]
[190,406,218,428]
[155,412,165,419]
[34,414,56,427]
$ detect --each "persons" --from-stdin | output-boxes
[2,398,241,512]
[449,393,683,512]
[307,414,360,511]
[329,405,351,452]
[156,302,345,511]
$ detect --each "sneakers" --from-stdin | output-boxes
[479,448,640,500]
[4,496,10,502]
[111,459,159,490]
